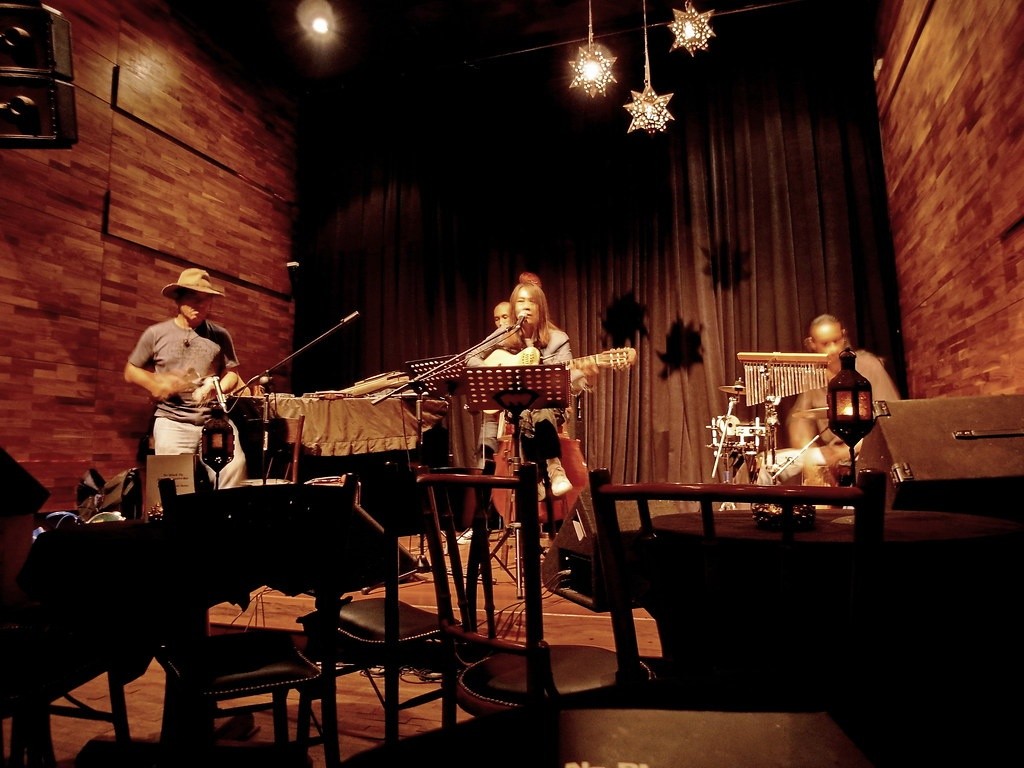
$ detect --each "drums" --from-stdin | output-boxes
[736,422,771,449]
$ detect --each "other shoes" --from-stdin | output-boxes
[456,528,473,544]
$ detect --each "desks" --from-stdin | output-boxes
[652,510,1023,570]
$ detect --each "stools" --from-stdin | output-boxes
[500,424,569,569]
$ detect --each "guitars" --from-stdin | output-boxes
[480,347,642,414]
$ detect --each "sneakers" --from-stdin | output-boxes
[547,459,573,497]
[538,478,545,502]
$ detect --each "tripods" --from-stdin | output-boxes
[361,325,519,593]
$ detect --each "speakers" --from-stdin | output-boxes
[347,504,419,591]
[542,486,701,614]
[853,394,1024,518]
[0,0,79,149]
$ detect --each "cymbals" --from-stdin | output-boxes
[719,382,747,395]
[791,407,834,421]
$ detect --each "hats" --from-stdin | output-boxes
[162,268,225,298]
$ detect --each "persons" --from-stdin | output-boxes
[121,267,249,521]
[0,446,51,607]
[785,314,898,486]
[470,271,600,524]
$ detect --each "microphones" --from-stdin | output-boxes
[515,312,528,330]
[212,376,227,412]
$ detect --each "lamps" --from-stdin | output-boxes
[201,406,235,490]
[828,347,875,482]
[569,0,716,134]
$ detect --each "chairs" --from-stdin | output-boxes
[153,472,359,767]
[590,469,885,710]
[0,519,149,768]
[296,460,497,767]
[414,461,619,727]
